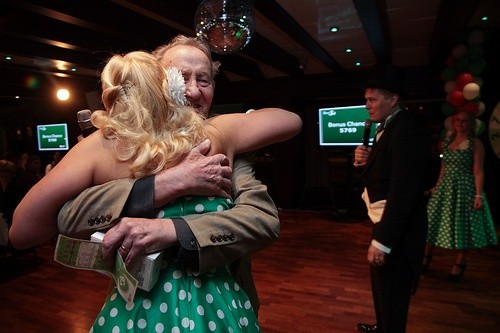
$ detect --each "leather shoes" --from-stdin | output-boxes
[357,323,377,333]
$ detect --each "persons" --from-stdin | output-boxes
[7,33,302,333]
[417,109,499,284]
[53,36,282,324]
[0,119,70,270]
[354,79,426,333]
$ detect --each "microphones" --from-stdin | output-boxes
[363,119,373,148]
[77,109,97,137]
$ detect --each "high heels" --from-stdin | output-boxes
[447,262,467,281]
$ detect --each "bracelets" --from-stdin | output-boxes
[474,195,484,198]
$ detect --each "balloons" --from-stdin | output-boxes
[438,55,486,137]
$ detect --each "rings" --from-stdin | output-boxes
[374,258,380,260]
[120,245,131,252]
